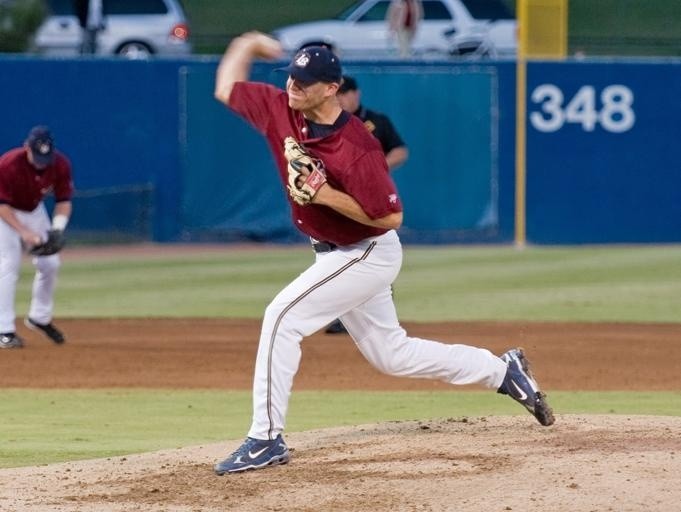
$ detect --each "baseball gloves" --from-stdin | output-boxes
[283,136,329,205]
[30,228,65,256]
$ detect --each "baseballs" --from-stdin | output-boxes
[34,235,43,245]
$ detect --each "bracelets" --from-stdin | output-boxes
[50,214,70,231]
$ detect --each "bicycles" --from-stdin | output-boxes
[422,18,503,62]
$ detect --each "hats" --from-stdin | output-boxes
[273,46,341,82]
[28,125,52,163]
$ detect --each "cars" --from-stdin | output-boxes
[267,1,518,65]
[31,1,193,61]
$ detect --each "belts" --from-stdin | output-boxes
[309,237,336,253]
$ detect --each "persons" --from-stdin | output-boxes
[294,41,342,59]
[72,0,110,55]
[314,76,408,333]
[214,30,549,477]
[2,126,74,353]
[387,0,425,60]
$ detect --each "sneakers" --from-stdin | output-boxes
[213,434,288,475]
[26,318,64,344]
[497,348,554,426]
[0,333,21,348]
